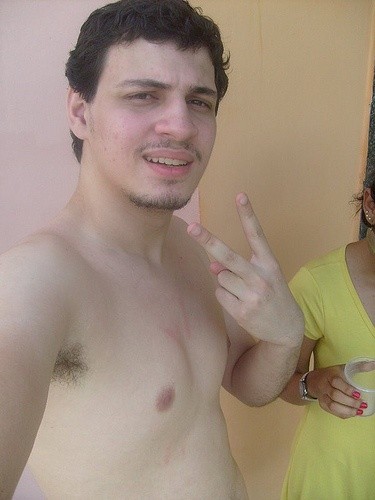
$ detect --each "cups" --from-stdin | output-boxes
[344,357,375,416]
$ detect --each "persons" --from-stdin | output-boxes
[0,0,306,500]
[279,167,375,500]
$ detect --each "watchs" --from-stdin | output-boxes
[300,372,318,401]
